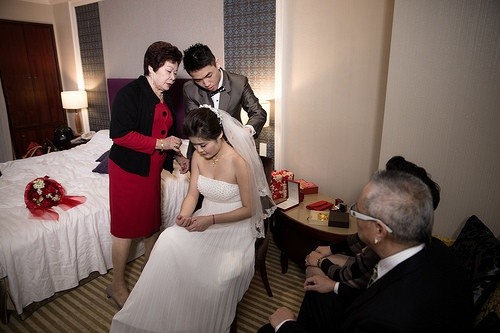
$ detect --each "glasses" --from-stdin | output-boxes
[348,201,394,234]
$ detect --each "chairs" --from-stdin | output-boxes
[448,215,500,333]
[251,190,273,298]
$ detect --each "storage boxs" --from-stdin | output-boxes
[275,181,300,211]
[294,180,319,194]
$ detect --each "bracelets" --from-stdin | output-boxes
[316,257,326,268]
[160,138,164,151]
[213,215,215,224]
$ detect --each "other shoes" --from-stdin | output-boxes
[106,287,123,309]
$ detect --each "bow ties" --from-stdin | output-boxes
[209,86,225,97]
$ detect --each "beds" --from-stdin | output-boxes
[0,79,191,315]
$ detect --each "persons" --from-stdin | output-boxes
[184,43,267,138]
[304,156,475,333]
[258,170,445,333]
[110,105,277,333]
[106,42,190,308]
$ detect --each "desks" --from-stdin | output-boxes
[275,191,360,275]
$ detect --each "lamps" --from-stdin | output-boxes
[240,101,270,127]
[61,91,88,138]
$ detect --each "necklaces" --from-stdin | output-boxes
[212,154,223,165]
[156,93,163,100]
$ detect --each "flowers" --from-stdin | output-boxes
[23,174,87,221]
[268,169,296,198]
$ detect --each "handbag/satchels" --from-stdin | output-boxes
[22,142,44,159]
[54,124,74,149]
